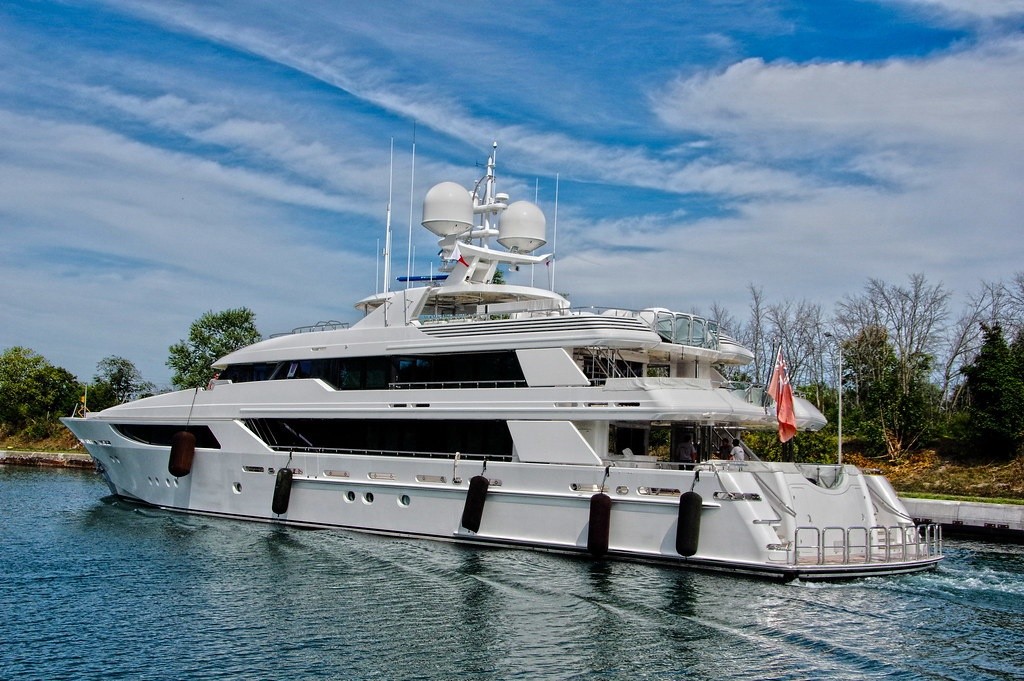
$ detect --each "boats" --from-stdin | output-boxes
[58,141,946,580]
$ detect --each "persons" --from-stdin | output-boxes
[720,438,744,461]
[676,435,704,470]
[207,372,219,390]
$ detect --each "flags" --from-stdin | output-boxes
[768,351,797,443]
[450,245,469,267]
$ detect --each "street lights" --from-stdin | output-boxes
[823,332,843,468]
